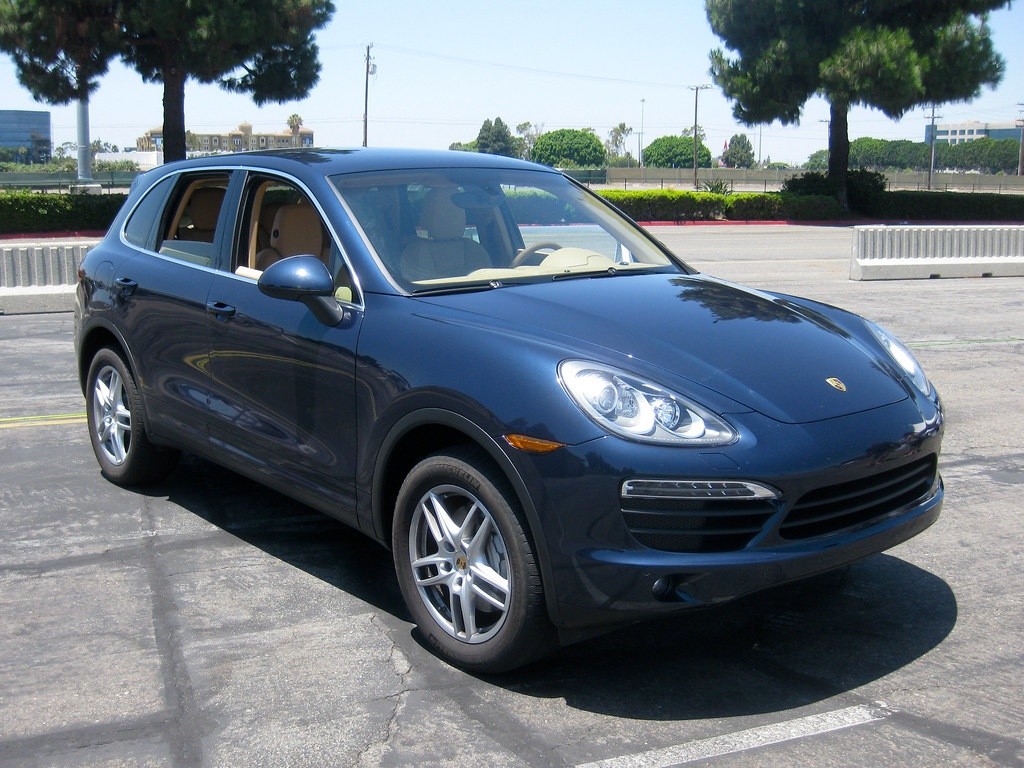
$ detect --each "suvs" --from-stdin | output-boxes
[73,146,947,671]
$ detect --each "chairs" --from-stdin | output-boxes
[252,206,351,288]
[405,186,491,278]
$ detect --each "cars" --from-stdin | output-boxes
[764,162,789,170]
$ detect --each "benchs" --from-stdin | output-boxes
[177,189,410,268]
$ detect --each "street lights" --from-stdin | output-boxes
[640,98,646,164]
